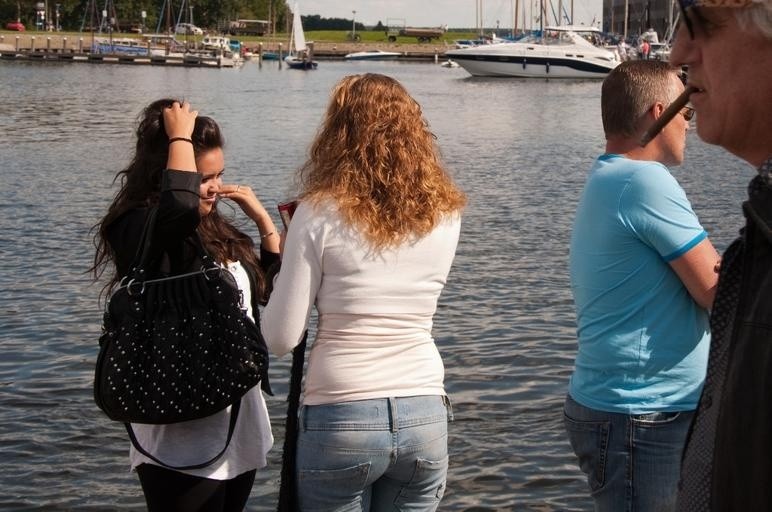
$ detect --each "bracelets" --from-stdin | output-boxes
[169,137,193,144]
[261,232,273,239]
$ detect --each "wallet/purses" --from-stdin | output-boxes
[278,194,306,233]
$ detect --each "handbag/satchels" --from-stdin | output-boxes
[94,200,270,425]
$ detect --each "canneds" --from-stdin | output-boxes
[278,196,297,230]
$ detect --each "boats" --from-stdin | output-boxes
[446,25,688,82]
[98,35,319,69]
[345,49,402,60]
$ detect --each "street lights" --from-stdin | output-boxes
[351,10,356,40]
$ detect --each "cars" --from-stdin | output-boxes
[173,23,203,36]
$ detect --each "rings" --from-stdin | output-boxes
[236,185,240,192]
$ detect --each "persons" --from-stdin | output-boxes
[260,74,461,512]
[100,99,281,512]
[564,61,722,512]
[617,36,651,62]
[670,0,772,512]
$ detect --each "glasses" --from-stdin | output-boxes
[662,100,696,121]
[676,0,758,40]
